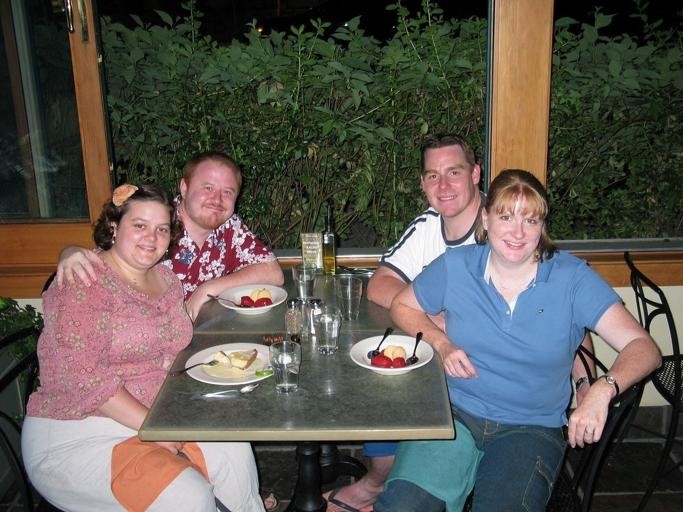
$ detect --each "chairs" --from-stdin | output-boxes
[0,324,64,512]
[538,326,655,511]
[602,251,682,511]
[40,270,56,295]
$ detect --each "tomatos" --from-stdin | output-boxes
[371,355,391,367]
[253,297,271,308]
[241,296,255,308]
[391,358,406,367]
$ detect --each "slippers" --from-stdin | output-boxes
[326,486,360,512]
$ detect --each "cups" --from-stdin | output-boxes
[331,278,362,322]
[269,341,301,396]
[312,305,343,355]
[291,264,317,296]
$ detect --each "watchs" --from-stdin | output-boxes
[597,375,619,398]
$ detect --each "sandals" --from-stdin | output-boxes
[259,486,281,512]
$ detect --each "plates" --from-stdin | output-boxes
[217,284,286,315]
[348,335,432,375]
[185,341,273,384]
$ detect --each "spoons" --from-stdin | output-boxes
[404,331,424,365]
[366,327,394,359]
[206,294,250,307]
[338,265,375,273]
[190,384,258,400]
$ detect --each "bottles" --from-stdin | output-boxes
[321,216,336,275]
[285,298,325,334]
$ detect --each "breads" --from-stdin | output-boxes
[214,348,257,370]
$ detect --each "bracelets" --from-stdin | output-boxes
[575,377,596,391]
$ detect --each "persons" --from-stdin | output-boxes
[21,183,267,512]
[373,170,662,512]
[322,135,596,512]
[57,151,284,511]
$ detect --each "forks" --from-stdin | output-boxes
[168,359,218,377]
